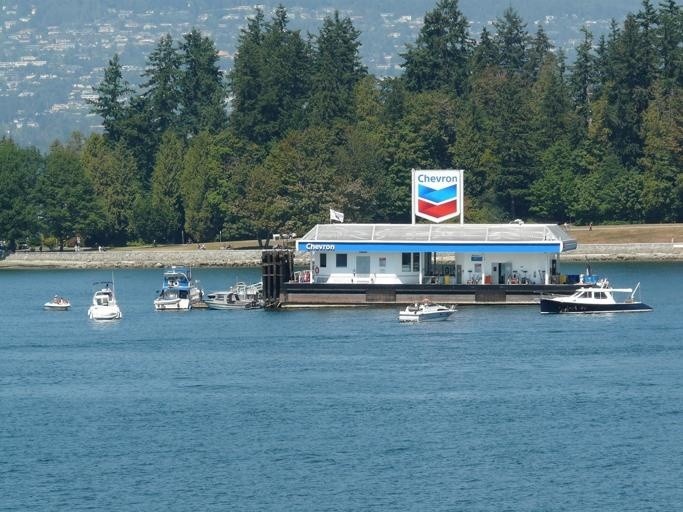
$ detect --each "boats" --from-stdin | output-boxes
[399,300,457,323]
[541,277,653,314]
[88,273,122,320]
[45,300,70,311]
[153,265,206,311]
[206,282,264,309]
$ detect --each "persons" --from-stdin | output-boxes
[74,244,77,252]
[101,245,105,252]
[223,243,231,250]
[98,245,101,252]
[77,244,80,251]
[590,223,592,231]
[197,242,206,249]
[53,295,68,305]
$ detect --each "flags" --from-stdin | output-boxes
[330,208,344,223]
